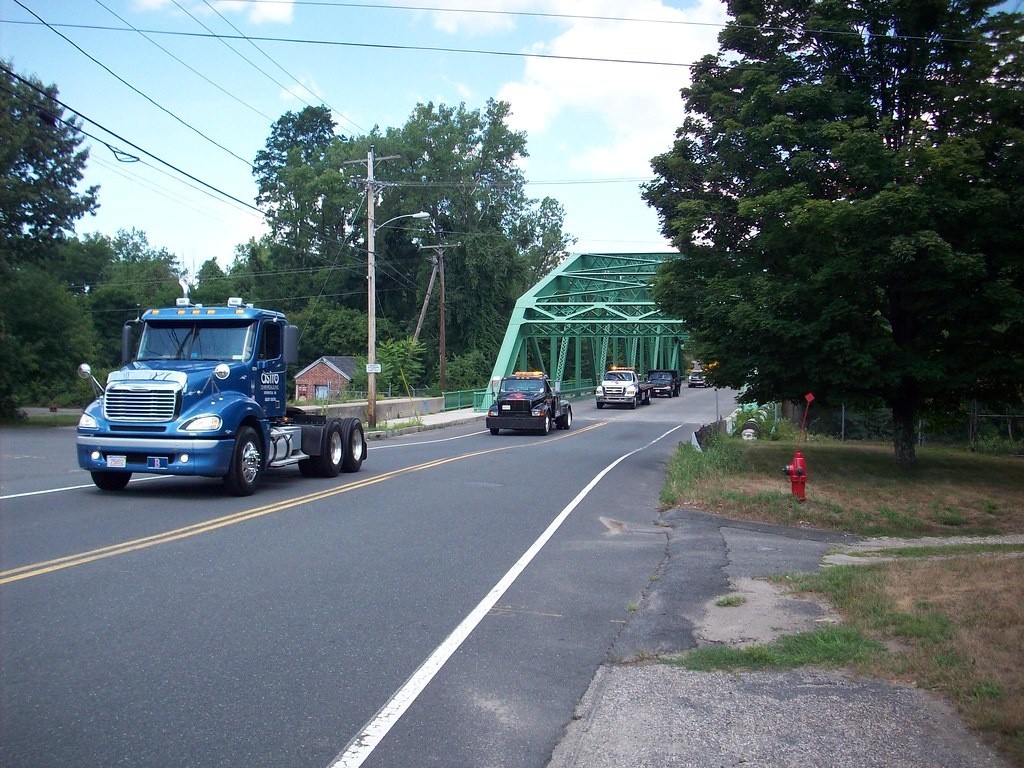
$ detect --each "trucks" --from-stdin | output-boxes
[644,369,681,397]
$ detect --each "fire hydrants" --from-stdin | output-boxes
[781,451,808,501]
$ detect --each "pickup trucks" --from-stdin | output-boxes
[595,366,654,409]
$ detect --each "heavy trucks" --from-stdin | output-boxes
[76,296,367,497]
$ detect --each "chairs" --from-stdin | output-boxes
[651,374,658,380]
[528,387,537,391]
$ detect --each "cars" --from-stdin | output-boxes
[688,370,713,387]
[486,371,572,435]
